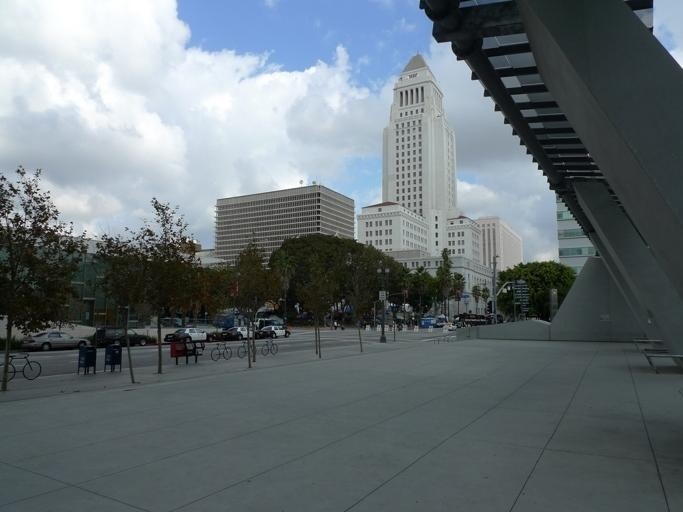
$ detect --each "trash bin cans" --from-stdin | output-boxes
[104,344,122,372]
[78,345,96,375]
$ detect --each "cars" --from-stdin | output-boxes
[22,323,294,352]
[159,312,278,329]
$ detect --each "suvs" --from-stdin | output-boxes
[459,313,485,328]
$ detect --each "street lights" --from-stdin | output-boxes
[376,268,390,343]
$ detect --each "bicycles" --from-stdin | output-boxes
[0,354,42,383]
[210,336,279,360]
